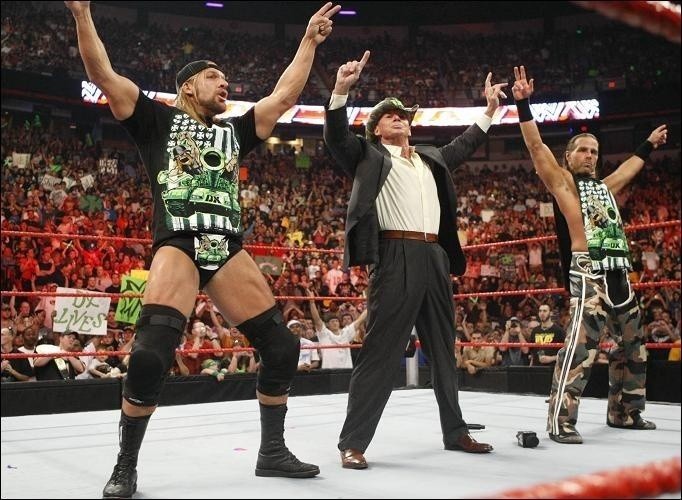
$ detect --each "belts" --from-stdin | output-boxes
[379,229,438,244]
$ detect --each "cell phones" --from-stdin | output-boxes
[201,327,206,335]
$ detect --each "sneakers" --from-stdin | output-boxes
[608,413,656,430]
[548,429,583,443]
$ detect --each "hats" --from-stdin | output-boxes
[366,97,419,144]
[173,60,221,101]
[61,329,80,339]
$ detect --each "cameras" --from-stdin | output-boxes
[511,322,517,328]
[655,323,660,327]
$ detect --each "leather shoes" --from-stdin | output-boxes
[339,448,368,469]
[445,431,493,453]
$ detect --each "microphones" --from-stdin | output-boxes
[516,430,539,448]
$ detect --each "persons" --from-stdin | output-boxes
[509,62,669,449]
[324,45,509,470]
[0,1,682,384]
[58,0,343,498]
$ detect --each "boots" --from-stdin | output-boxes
[102,409,151,499]
[255,401,320,478]
